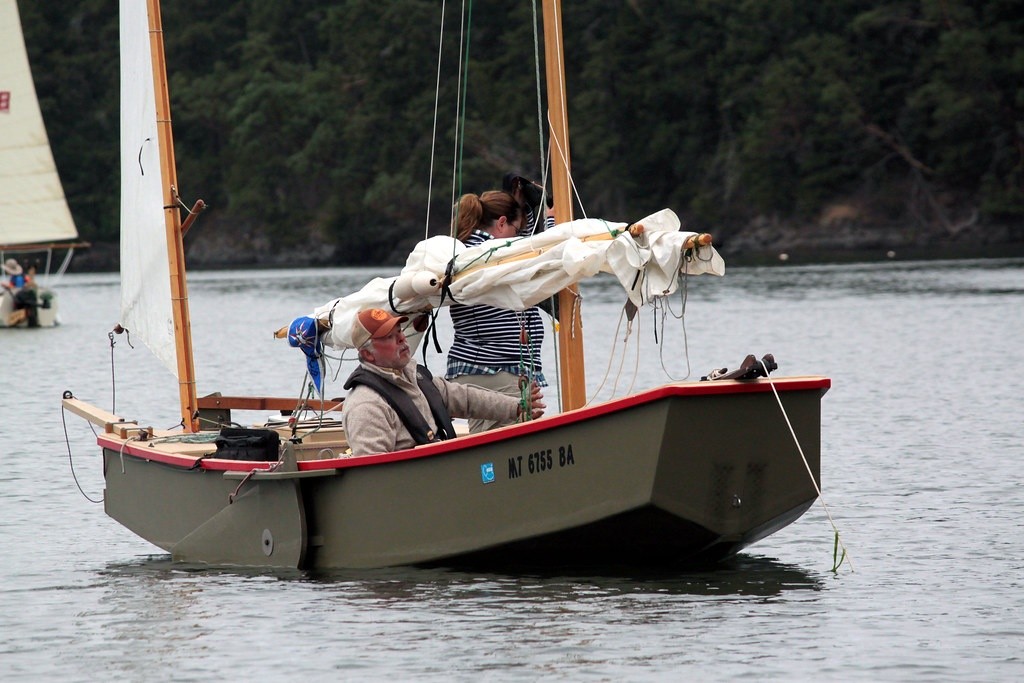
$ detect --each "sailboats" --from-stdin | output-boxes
[61,0,833,574]
[0,0,91,328]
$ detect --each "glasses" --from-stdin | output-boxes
[508,221,523,236]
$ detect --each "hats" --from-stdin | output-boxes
[1,258,23,275]
[352,308,409,350]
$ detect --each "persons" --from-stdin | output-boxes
[446,190,555,434]
[343,308,547,460]
[0,258,31,290]
